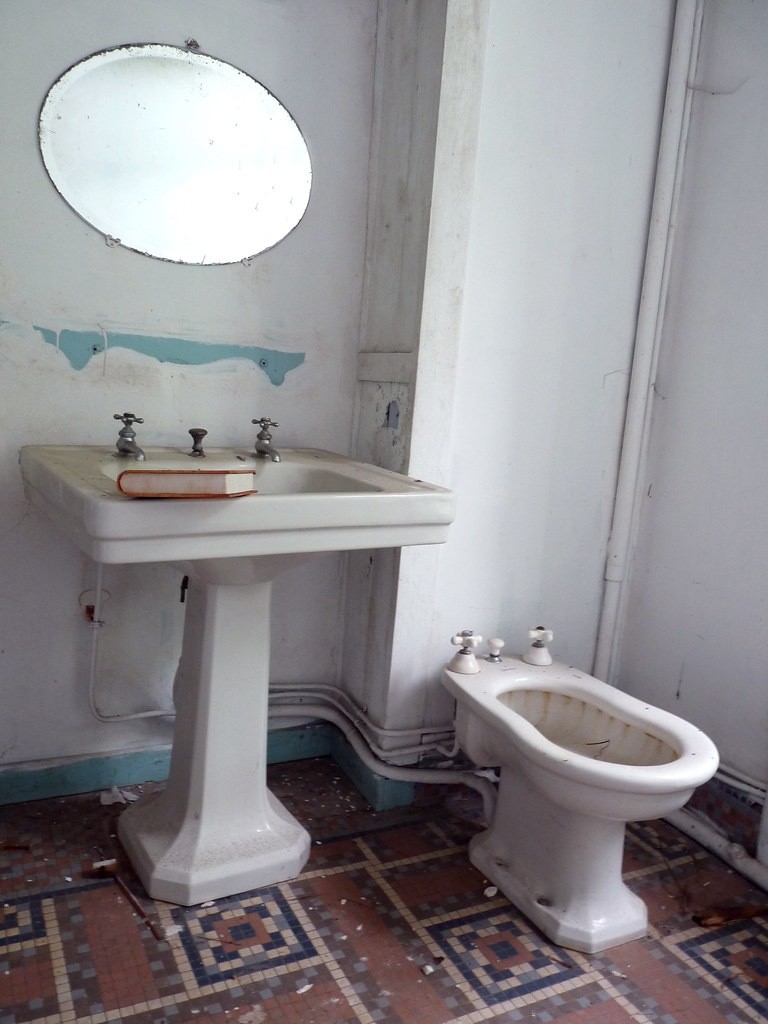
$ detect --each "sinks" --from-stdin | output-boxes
[16,440,457,565]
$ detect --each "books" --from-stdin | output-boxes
[117,470,259,498]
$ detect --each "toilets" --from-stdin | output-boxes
[441,645,722,954]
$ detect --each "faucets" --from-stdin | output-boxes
[252,417,281,463]
[113,411,145,461]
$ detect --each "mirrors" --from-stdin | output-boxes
[39,43,313,266]
